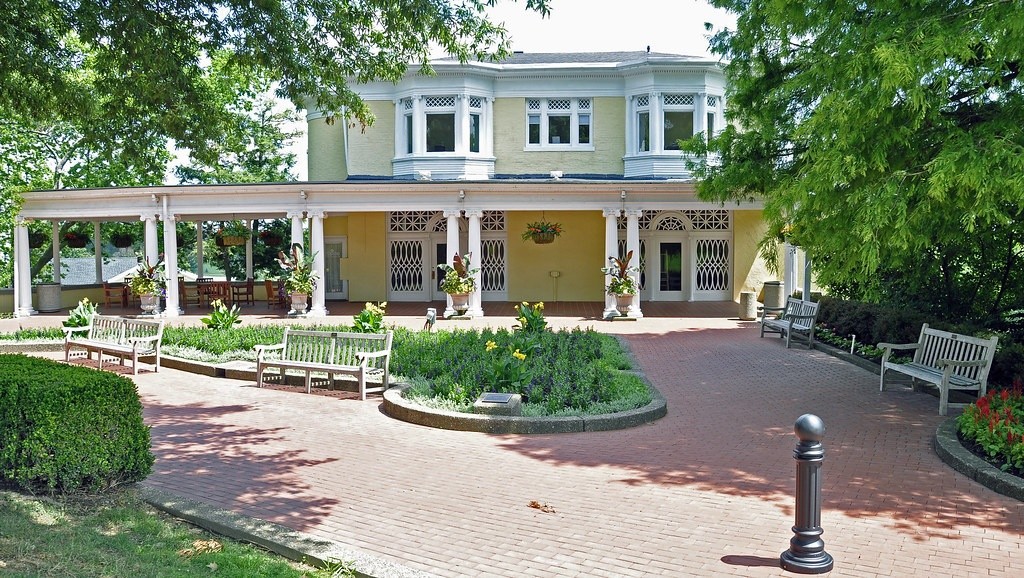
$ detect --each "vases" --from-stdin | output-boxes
[534,232,554,242]
[221,236,246,246]
[67,237,87,248]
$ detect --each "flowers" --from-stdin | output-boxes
[520,221,564,242]
[62,230,90,243]
[216,220,250,241]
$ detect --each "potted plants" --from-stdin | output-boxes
[603,275,638,316]
[259,231,284,246]
[130,276,164,314]
[27,229,50,248]
[440,271,478,316]
[109,232,137,248]
[284,270,316,312]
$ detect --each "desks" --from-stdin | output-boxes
[197,282,255,307]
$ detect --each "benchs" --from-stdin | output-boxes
[757,295,822,351]
[876,323,998,416]
[252,325,394,401]
[61,314,165,376]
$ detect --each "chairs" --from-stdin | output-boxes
[103,276,188,312]
[264,280,287,311]
[196,278,255,308]
